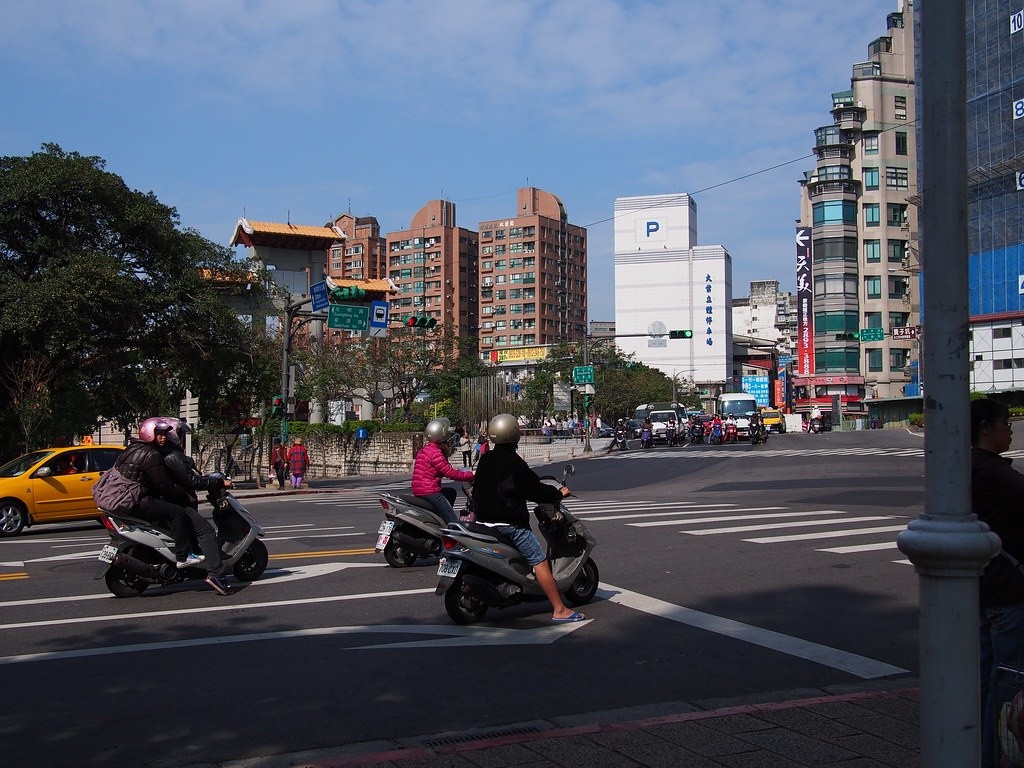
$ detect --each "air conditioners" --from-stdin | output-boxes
[522,250,527,253]
[425,243,429,247]
[484,250,488,253]
[528,250,530,253]
[430,255,434,258]
[395,263,398,267]
[555,255,574,304]
[427,304,430,307]
[847,139,854,144]
[478,325,482,328]
[485,282,491,287]
[492,309,496,313]
[391,278,394,281]
[430,239,434,245]
[430,267,434,270]
[481,284,484,287]
[395,305,399,309]
[531,249,534,253]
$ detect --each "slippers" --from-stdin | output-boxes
[552,610,585,622]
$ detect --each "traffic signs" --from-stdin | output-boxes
[860,328,885,341]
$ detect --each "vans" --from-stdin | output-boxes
[716,392,758,441]
[621,401,714,442]
[647,409,682,444]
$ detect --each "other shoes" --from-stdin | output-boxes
[277,486,284,490]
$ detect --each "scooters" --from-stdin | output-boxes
[747,416,769,445]
[709,420,726,445]
[435,463,600,625]
[689,419,705,445]
[808,415,833,434]
[639,424,654,449]
[93,458,269,599]
[611,427,626,451]
[374,451,480,569]
[664,422,678,447]
[723,422,738,444]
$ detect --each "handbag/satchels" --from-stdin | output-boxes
[269,468,277,479]
[92,462,141,511]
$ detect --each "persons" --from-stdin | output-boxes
[287,438,310,489]
[640,416,656,448]
[596,414,602,437]
[723,413,740,444]
[665,418,680,446]
[480,436,489,454]
[707,414,723,444]
[971,397,1024,768]
[688,414,705,443]
[609,419,630,450]
[454,424,463,440]
[807,405,821,433]
[114,417,206,569]
[459,431,472,467]
[473,435,484,469]
[542,414,574,444]
[471,414,586,621]
[161,417,233,595]
[870,416,882,429]
[270,438,288,490]
[58,454,78,475]
[749,411,765,434]
[284,444,291,478]
[411,418,476,524]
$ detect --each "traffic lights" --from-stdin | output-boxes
[693,390,707,395]
[403,315,437,329]
[271,395,285,417]
[624,361,632,369]
[584,394,592,408]
[669,329,693,339]
[835,332,860,342]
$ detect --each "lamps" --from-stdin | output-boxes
[664,245,666,250]
[638,247,641,252]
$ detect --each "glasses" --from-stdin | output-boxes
[1002,421,1012,429]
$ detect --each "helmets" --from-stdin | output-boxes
[729,413,733,419]
[140,417,173,443]
[645,417,650,423]
[161,418,193,446]
[618,419,623,425]
[692,414,700,418]
[753,413,756,418]
[669,418,673,423]
[757,412,760,417]
[425,417,451,443]
[488,414,520,444]
[814,405,819,409]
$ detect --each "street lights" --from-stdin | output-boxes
[672,368,700,401]
[709,377,733,414]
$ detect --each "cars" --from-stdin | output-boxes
[573,419,614,438]
[0,435,128,538]
[687,414,712,437]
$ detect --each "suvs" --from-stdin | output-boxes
[760,407,786,434]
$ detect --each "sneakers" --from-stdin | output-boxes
[176,550,206,569]
[205,574,228,596]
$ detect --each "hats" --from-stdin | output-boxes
[294,438,302,445]
[274,438,281,444]
